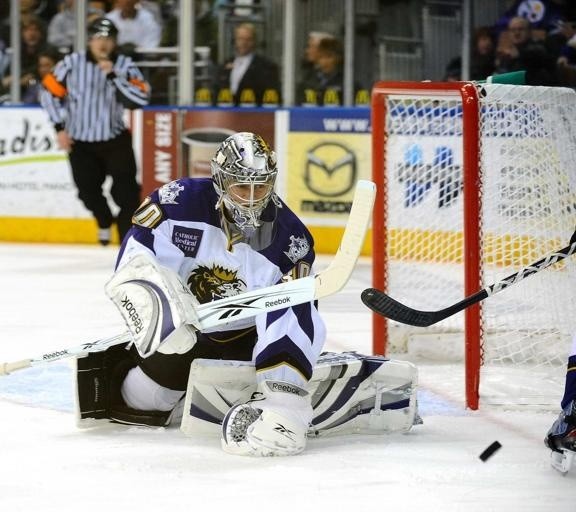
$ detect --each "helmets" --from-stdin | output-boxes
[87,17,118,40]
[209,132,283,232]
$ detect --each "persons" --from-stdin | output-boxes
[73,131,424,458]
[37,16,152,245]
[544,231,576,475]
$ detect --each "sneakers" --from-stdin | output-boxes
[99,228,110,246]
[544,398,576,449]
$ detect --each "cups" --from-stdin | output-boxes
[181,127,242,180]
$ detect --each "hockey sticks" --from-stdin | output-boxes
[359,242,575,327]
[0,181,377,375]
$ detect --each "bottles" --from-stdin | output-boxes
[486,70,527,87]
